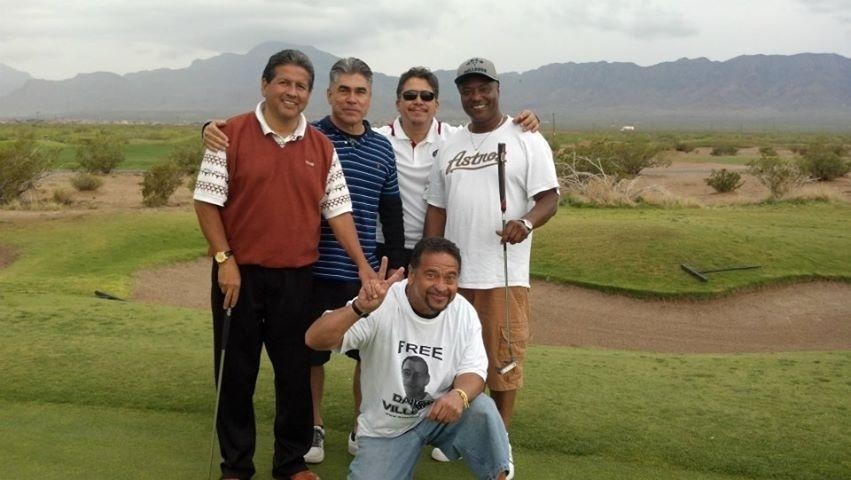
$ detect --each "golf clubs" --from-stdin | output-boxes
[495,144,517,375]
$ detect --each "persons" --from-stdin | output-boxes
[193,50,378,480]
[373,67,540,283]
[303,237,515,479]
[424,57,560,463]
[201,57,405,463]
[399,357,433,411]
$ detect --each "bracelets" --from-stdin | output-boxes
[535,115,539,122]
[455,389,469,411]
[202,121,211,139]
[352,300,369,319]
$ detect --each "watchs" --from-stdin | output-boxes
[215,250,233,263]
[519,219,533,231]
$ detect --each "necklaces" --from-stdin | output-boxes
[467,116,506,153]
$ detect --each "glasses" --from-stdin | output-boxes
[398,90,434,103]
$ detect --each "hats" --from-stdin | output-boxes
[454,57,500,83]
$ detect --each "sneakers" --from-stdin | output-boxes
[303,424,326,464]
